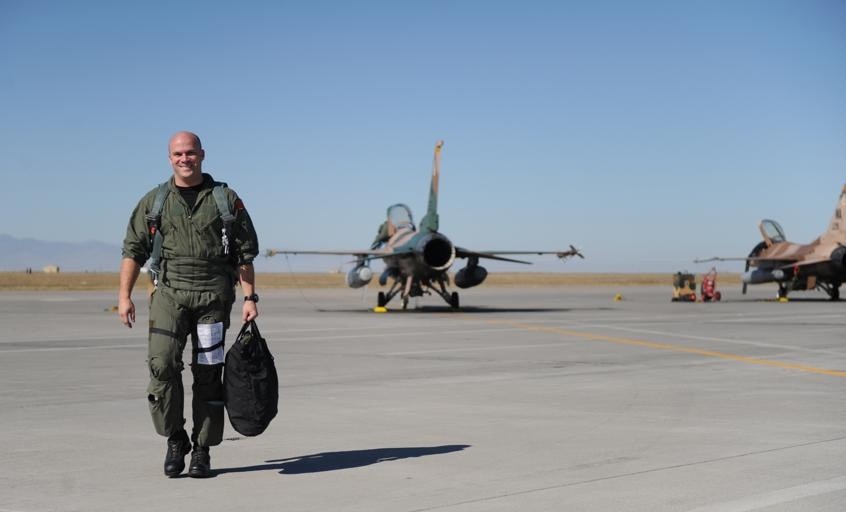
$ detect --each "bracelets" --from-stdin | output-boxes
[243,294,258,303]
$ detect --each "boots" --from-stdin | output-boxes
[163,430,192,478]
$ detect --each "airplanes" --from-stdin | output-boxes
[690,182,846,305]
[260,139,584,313]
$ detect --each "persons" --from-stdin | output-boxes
[118,130,261,478]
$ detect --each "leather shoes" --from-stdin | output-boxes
[188,445,211,478]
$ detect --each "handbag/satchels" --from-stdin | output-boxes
[223,320,279,437]
